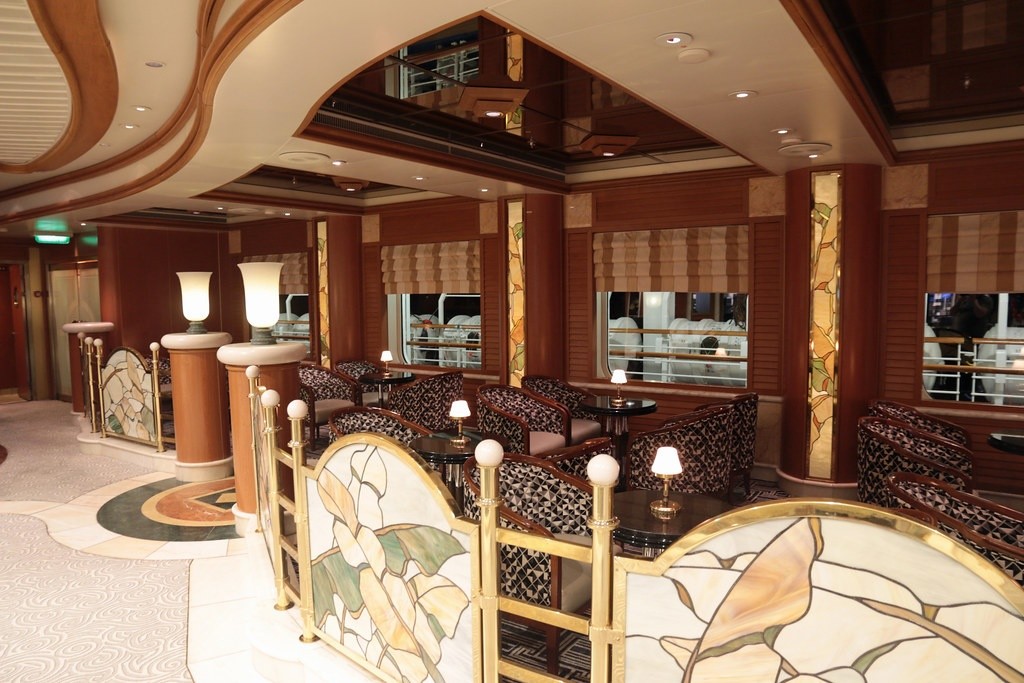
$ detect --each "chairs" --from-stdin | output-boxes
[666,393,759,502]
[886,471,1024,590]
[629,404,734,499]
[857,416,973,509]
[298,364,356,451]
[335,360,391,405]
[520,374,603,447]
[533,438,612,486]
[388,370,463,431]
[476,383,568,456]
[868,399,970,451]
[462,452,593,676]
[329,406,442,481]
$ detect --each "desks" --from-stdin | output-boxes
[577,396,658,492]
[410,430,510,513]
[612,492,750,560]
[359,371,416,407]
[988,430,1024,456]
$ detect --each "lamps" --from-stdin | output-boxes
[237,262,284,344]
[610,369,628,406]
[650,446,683,516]
[448,400,472,445]
[177,272,213,334]
[380,350,393,375]
